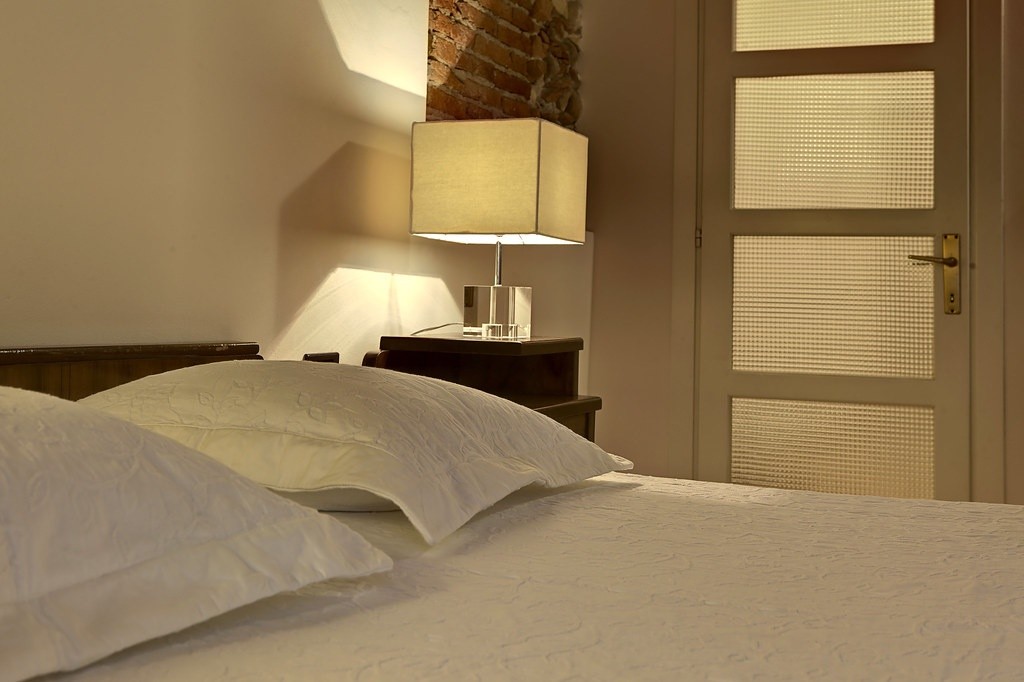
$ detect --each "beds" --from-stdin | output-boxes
[0,340,1024,682]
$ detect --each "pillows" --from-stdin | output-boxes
[74,360,634,552]
[0,386,394,682]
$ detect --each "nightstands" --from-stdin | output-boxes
[361,335,603,447]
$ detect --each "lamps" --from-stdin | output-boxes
[411,118,591,344]
